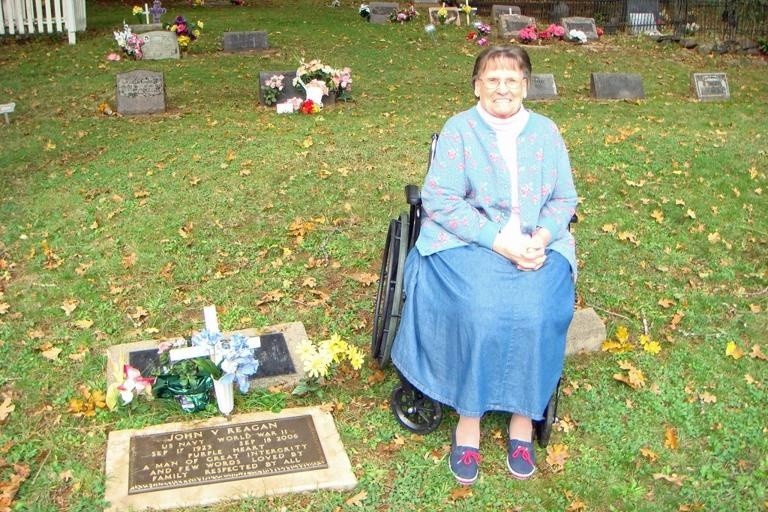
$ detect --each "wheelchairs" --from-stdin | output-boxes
[370,132,578,450]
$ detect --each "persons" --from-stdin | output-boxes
[390,46,577,484]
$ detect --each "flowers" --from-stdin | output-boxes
[97,104,112,115]
[107,0,205,61]
[360,4,604,44]
[264,58,354,112]
[106,330,366,415]
[230,0,245,6]
[655,7,700,37]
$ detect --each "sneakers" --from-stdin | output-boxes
[507,432,536,478]
[449,430,481,483]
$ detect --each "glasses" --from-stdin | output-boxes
[477,75,522,89]
